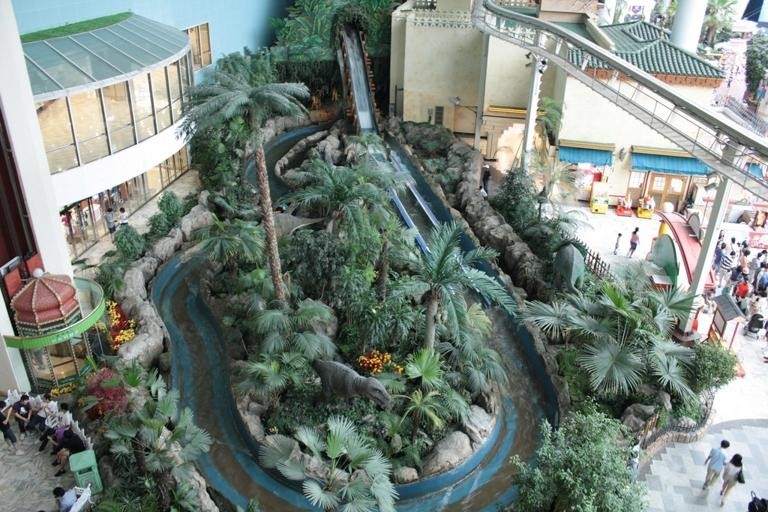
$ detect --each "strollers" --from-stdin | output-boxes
[742,311,766,341]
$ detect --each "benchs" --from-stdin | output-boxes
[4,389,93,450]
[69,483,95,512]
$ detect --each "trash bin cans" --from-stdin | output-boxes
[68,449,104,497]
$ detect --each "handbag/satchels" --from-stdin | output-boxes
[737,471,744,483]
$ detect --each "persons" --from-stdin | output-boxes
[0,394,85,477]
[53,487,78,512]
[482,165,492,193]
[719,454,744,507]
[712,230,768,343]
[106,208,117,244]
[118,208,128,226]
[480,186,488,200]
[614,233,622,255]
[626,227,640,258]
[702,440,730,490]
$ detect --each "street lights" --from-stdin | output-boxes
[535,185,549,222]
